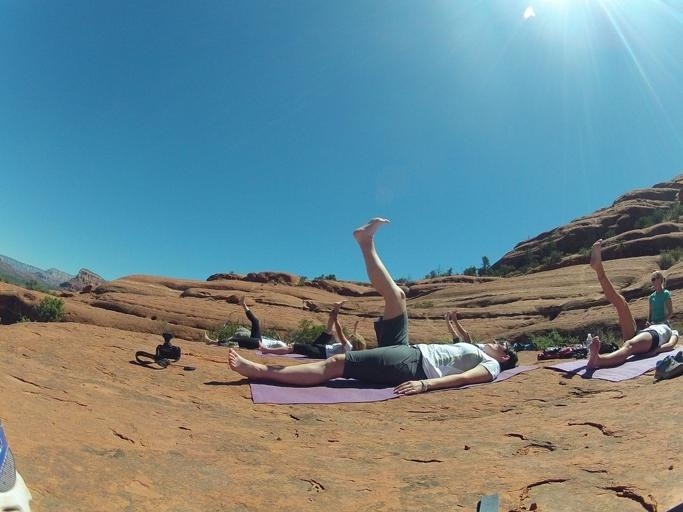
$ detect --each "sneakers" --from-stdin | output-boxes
[654,351,683,380]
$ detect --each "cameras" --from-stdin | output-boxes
[155,331,181,361]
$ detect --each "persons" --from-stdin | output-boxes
[646,271,674,326]
[203,294,288,350]
[227,216,520,396]
[259,300,366,359]
[443,309,497,350]
[583,236,680,372]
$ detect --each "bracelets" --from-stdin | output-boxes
[420,379,428,392]
